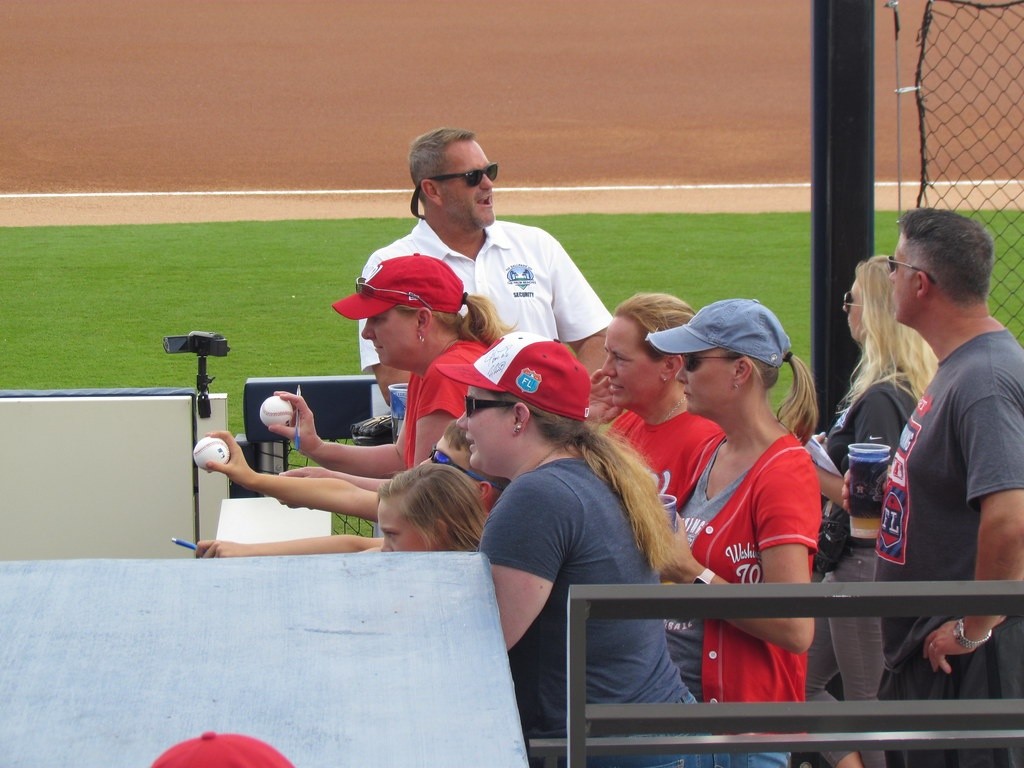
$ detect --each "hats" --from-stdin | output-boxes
[645,299,791,368]
[332,253,464,320]
[434,332,592,422]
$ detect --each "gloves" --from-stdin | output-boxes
[350,415,392,446]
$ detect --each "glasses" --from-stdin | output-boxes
[888,256,937,285]
[681,353,744,372]
[356,276,433,311]
[422,162,498,186]
[428,444,504,491]
[842,291,862,314]
[464,395,545,418]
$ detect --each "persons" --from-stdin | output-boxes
[873,209,1024,768]
[261,253,499,510]
[377,467,486,557]
[189,420,510,559]
[432,329,712,768]
[587,288,725,529]
[801,253,940,768]
[418,418,510,512]
[648,297,821,768]
[359,128,622,405]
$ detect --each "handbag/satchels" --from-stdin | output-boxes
[814,522,850,573]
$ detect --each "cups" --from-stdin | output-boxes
[388,383,408,444]
[848,444,891,539]
[657,495,677,585]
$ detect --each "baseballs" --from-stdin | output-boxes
[193,437,230,472]
[259,396,294,427]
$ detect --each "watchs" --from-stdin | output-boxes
[953,618,992,649]
[692,568,717,585]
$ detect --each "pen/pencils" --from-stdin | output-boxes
[295,384,303,452]
[172,537,197,551]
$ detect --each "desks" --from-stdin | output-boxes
[0,548,531,768]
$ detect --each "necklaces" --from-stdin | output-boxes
[663,392,687,423]
